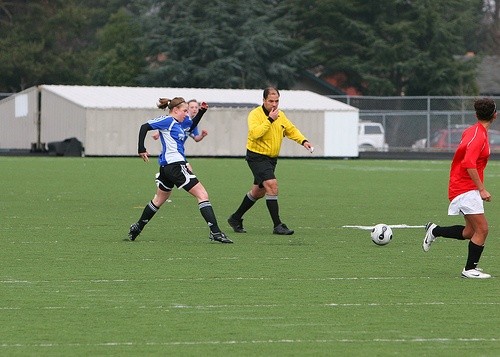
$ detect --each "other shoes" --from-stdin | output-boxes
[155,173,160,187]
[153,195,172,203]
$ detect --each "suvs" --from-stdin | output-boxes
[359,122,388,152]
[412,123,500,148]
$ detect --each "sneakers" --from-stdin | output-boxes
[227,214,246,233]
[209,231,233,244]
[273,224,294,235]
[128,224,141,241]
[422,222,437,252]
[461,267,491,279]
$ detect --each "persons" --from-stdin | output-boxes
[422,95,498,278]
[227,86,314,234]
[152,99,209,187]
[127,95,235,246]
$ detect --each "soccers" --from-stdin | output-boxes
[371,224,392,246]
